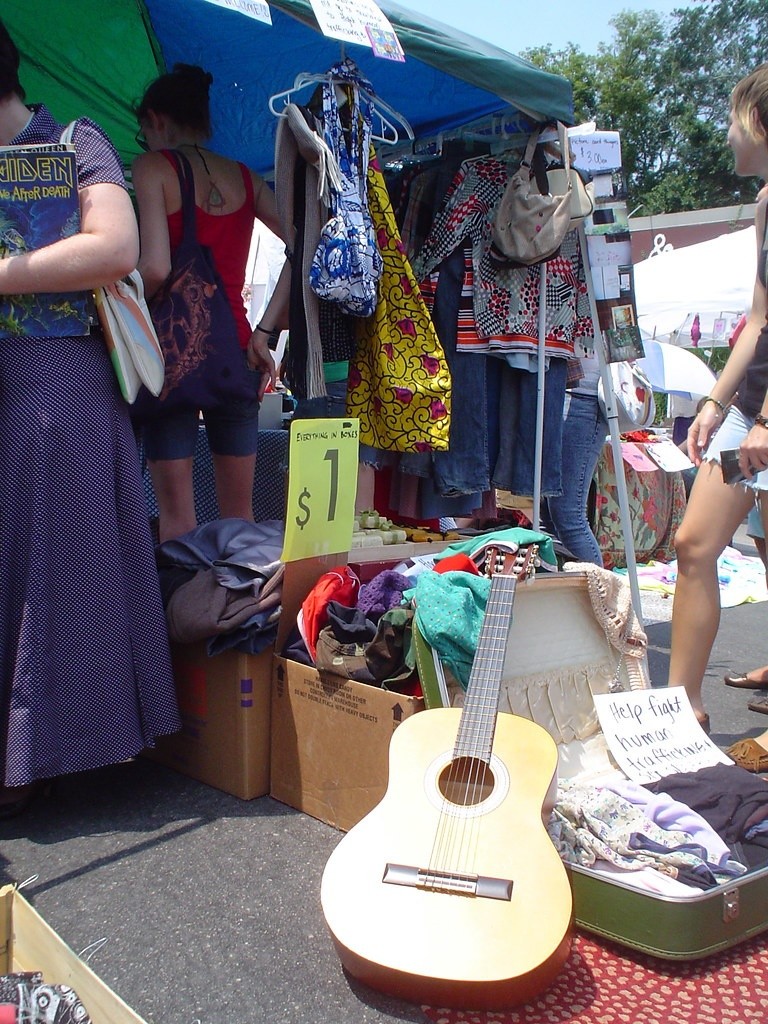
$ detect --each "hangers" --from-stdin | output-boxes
[269,42,576,172]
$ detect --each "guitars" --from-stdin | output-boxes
[320,543,575,1010]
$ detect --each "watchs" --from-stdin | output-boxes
[696,395,727,415]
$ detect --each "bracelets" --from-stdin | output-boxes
[755,413,768,426]
[257,326,273,336]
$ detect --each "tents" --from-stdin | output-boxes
[641,338,718,399]
[1,0,644,637]
[632,218,768,349]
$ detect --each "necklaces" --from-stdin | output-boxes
[176,142,214,177]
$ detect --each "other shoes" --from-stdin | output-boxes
[724,673,768,689]
[748,698,768,714]
[725,738,768,774]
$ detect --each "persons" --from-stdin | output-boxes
[132,63,291,543]
[547,337,609,577]
[0,23,183,820]
[661,63,768,774]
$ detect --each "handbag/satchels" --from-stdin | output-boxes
[528,166,593,230]
[597,361,657,432]
[145,151,261,406]
[492,121,573,266]
[57,122,166,406]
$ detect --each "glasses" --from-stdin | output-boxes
[134,127,154,152]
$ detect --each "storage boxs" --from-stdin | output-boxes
[269,652,426,834]
[159,629,273,800]
[0,883,148,1024]
